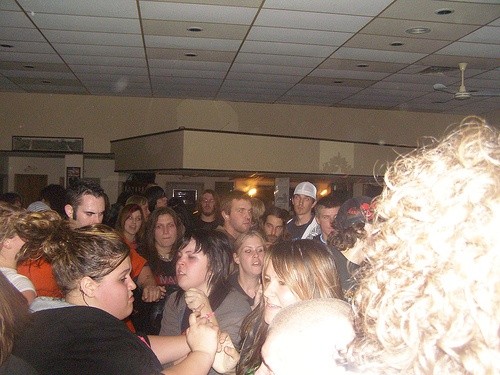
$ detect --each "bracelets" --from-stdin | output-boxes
[203,312,216,319]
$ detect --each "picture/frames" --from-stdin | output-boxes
[172,188,197,212]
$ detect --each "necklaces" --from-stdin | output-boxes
[159,252,175,263]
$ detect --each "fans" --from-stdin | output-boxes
[429,62,500,104]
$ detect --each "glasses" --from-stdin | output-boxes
[202,198,214,203]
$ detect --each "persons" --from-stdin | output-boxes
[0,173,374,375]
[342,124,500,375]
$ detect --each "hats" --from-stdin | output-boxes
[27,201,51,211]
[293,181,317,199]
[336,195,376,227]
